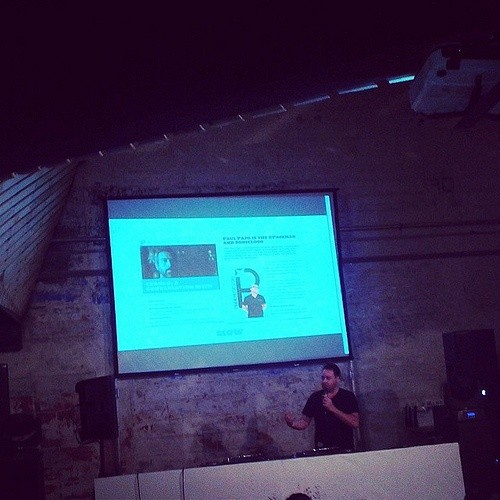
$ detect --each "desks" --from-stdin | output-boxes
[97,442,465,500]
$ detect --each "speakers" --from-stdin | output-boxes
[75,374,119,442]
[442,328,500,400]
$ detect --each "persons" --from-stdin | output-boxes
[282,362,362,449]
[154,248,178,278]
[241,284,266,318]
